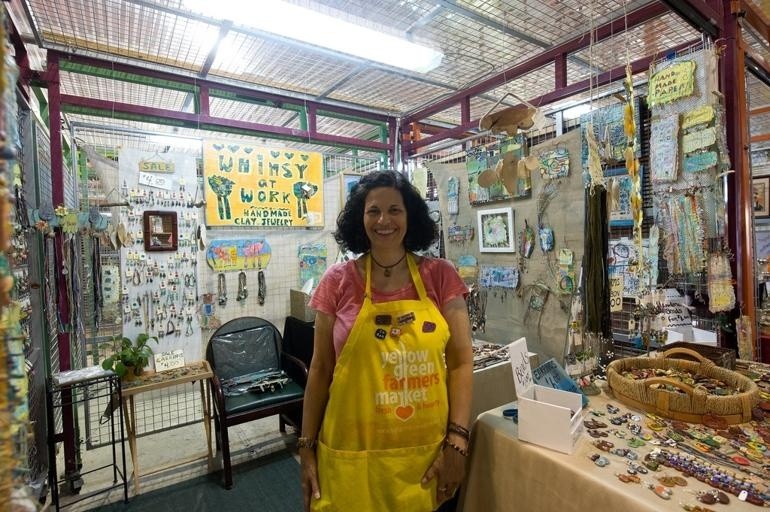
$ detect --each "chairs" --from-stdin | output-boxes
[204,316,310,490]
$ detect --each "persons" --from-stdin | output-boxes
[296,170,474,512]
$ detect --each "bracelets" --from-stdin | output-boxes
[298,437,318,448]
[449,419,472,442]
[447,440,470,458]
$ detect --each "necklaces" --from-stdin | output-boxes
[370,253,407,277]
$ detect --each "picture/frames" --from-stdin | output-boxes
[476,207,516,254]
[144,210,178,251]
[751,175,770,221]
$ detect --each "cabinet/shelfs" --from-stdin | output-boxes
[279,316,316,434]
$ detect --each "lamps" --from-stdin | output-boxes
[180,1,448,81]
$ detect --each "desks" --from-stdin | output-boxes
[456,346,770,511]
[116,360,215,495]
[44,363,127,512]
[473,338,539,429]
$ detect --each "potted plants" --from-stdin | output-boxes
[99,332,160,382]
